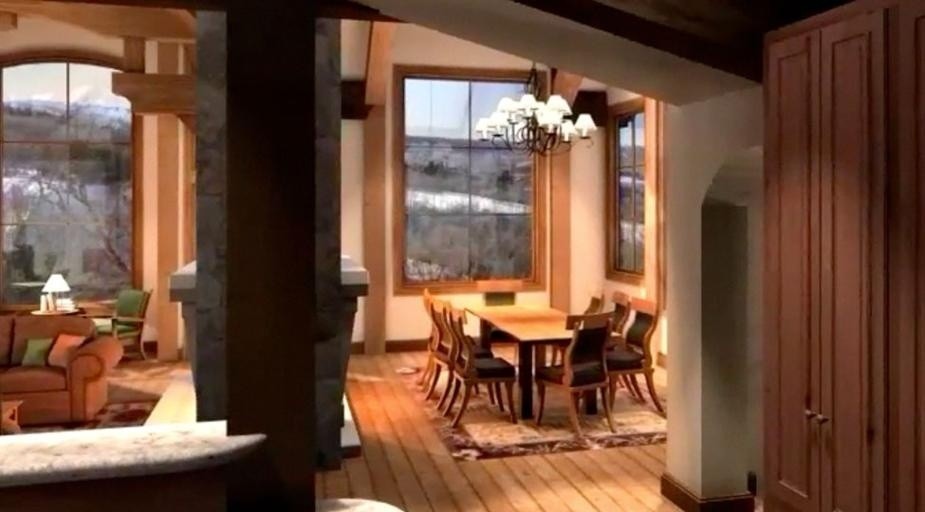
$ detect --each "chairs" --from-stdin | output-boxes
[426,301,502,409]
[592,295,667,423]
[531,305,618,441]
[482,290,521,367]
[416,289,480,398]
[95,286,156,363]
[442,305,522,431]
[601,294,633,350]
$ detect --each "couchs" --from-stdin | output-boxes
[0,308,127,427]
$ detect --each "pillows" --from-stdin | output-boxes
[47,334,87,372]
[19,337,57,371]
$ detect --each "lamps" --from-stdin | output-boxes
[472,55,599,159]
[39,271,71,306]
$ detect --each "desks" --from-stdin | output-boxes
[464,302,623,423]
[33,304,86,322]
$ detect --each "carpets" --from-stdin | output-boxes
[404,362,669,462]
[18,395,158,433]
